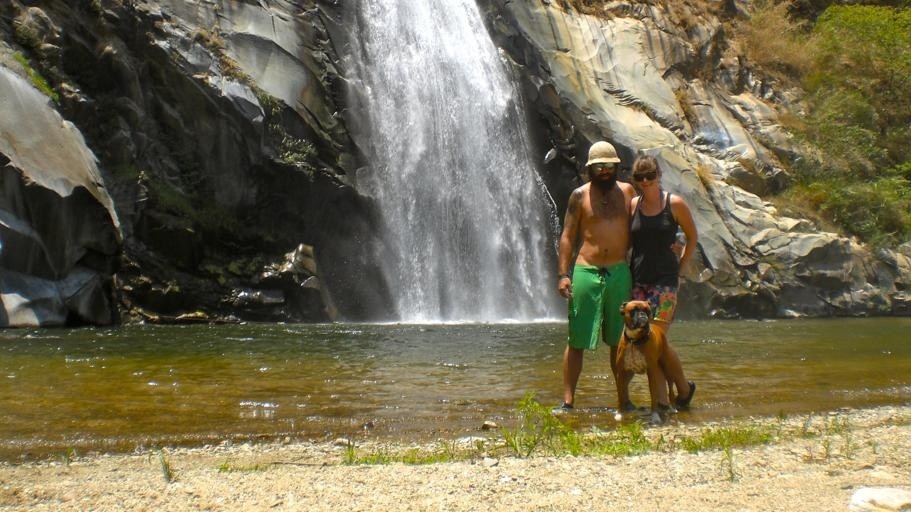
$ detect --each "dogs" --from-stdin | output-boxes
[614,297,677,413]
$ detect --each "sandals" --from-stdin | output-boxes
[676,379,696,408]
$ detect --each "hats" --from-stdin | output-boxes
[583,139,622,167]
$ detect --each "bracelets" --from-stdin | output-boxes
[559,275,570,280]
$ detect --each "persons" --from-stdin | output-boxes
[626,153,701,413]
[556,139,686,414]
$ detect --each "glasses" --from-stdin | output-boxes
[632,171,657,182]
[589,162,615,172]
